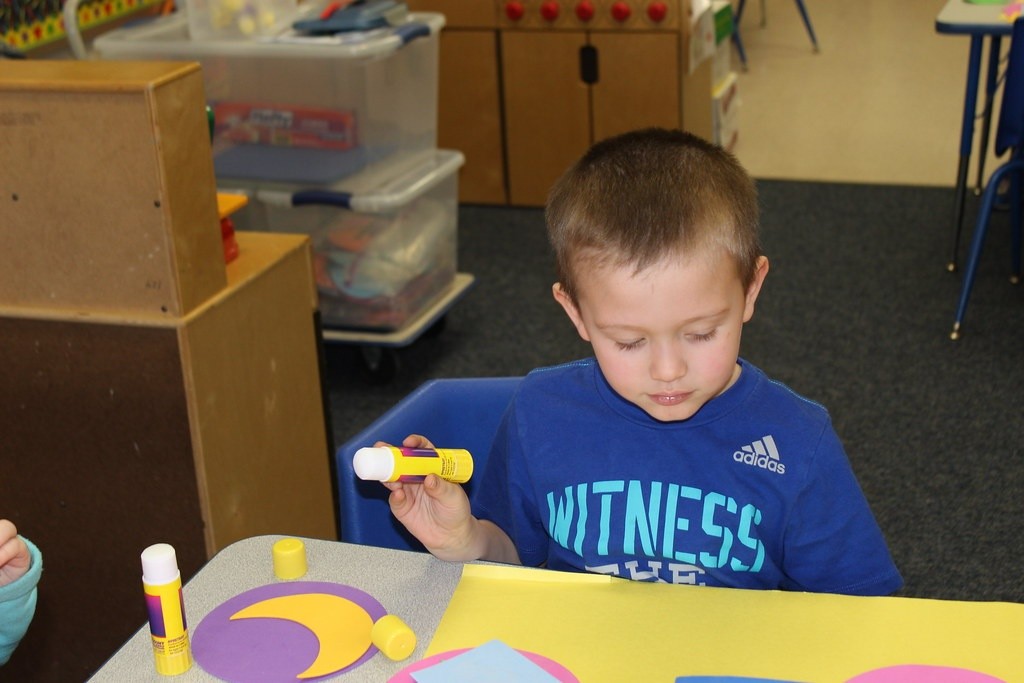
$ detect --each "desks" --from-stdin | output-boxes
[87,534,1024,683]
[935,0,1024,284]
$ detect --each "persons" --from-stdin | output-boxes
[0,519,43,671]
[372,127,905,600]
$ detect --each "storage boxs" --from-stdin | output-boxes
[93,11,446,193]
[186,0,296,41]
[258,150,466,334]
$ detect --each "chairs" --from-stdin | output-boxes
[944,16,1024,343]
[335,377,523,553]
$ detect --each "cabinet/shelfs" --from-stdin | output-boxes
[397,0,714,207]
